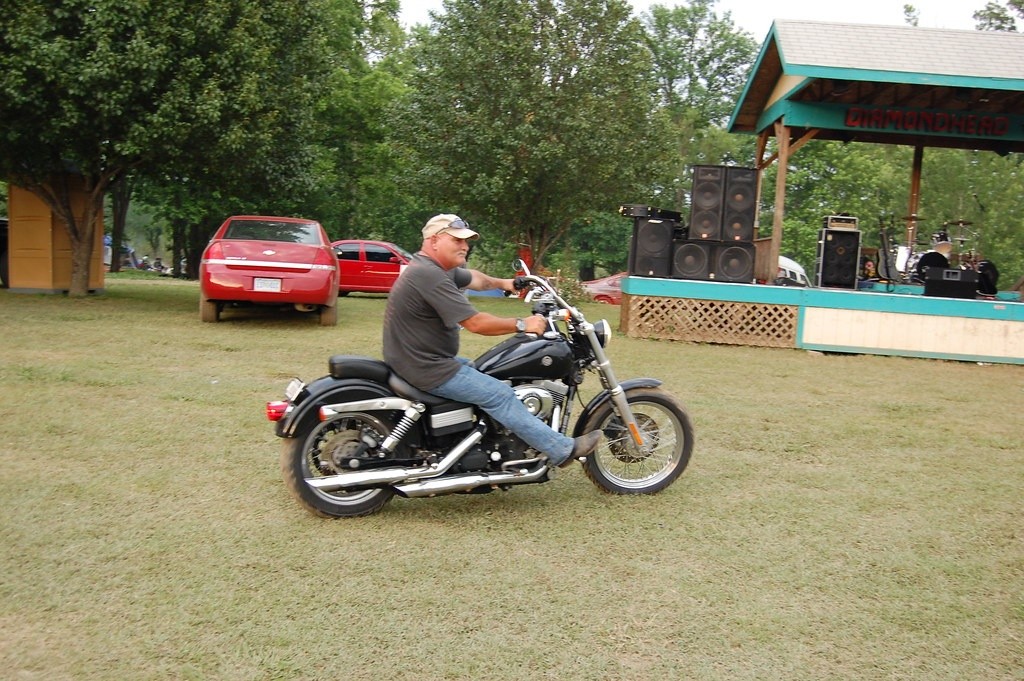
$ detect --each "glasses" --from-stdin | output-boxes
[436,220,470,235]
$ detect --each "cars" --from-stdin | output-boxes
[580,271,630,306]
[331,240,414,297]
[200,216,343,326]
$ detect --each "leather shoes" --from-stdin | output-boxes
[557,429,603,468]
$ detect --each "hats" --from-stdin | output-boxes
[421,214,480,240]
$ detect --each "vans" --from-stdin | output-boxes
[774,255,812,287]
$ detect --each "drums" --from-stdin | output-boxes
[905,250,949,283]
[930,231,953,253]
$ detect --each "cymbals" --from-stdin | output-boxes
[902,216,926,222]
[950,220,974,226]
[954,238,970,242]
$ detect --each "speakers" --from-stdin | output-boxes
[673,238,756,284]
[626,216,675,279]
[721,166,759,243]
[924,267,977,300]
[814,228,862,291]
[688,165,726,241]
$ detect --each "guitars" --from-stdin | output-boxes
[875,220,899,280]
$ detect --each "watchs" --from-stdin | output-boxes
[515,317,526,333]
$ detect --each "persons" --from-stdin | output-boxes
[382,213,604,469]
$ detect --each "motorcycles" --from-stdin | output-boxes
[268,259,693,521]
